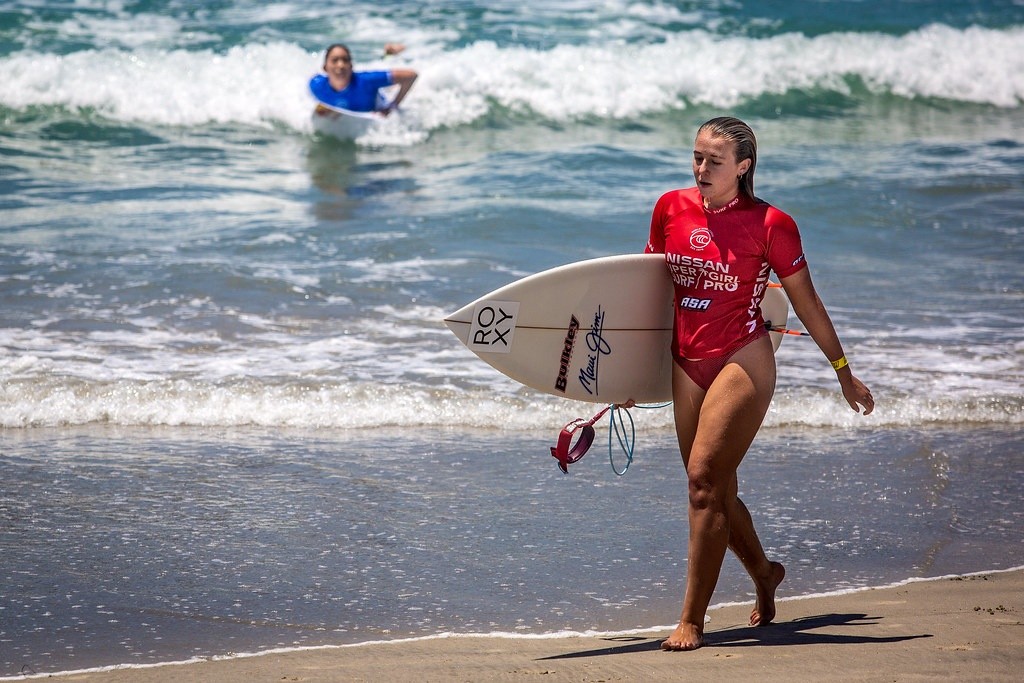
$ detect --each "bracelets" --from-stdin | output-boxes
[830,356,849,371]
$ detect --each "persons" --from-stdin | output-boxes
[310,44,418,120]
[643,117,874,651]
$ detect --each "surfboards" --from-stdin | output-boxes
[442,252,791,404]
[310,102,385,139]
